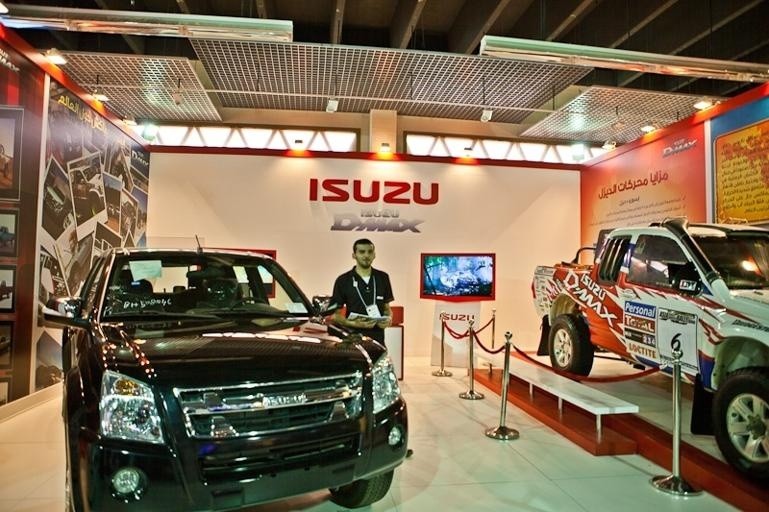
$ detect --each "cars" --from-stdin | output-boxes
[40,241,413,512]
[71,166,105,202]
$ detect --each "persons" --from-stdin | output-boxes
[326,239,415,459]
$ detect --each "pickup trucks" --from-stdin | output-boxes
[530,216,769,489]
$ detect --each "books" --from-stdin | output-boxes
[347,311,390,324]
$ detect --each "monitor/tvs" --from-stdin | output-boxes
[420,252,495,303]
[198,247,276,298]
